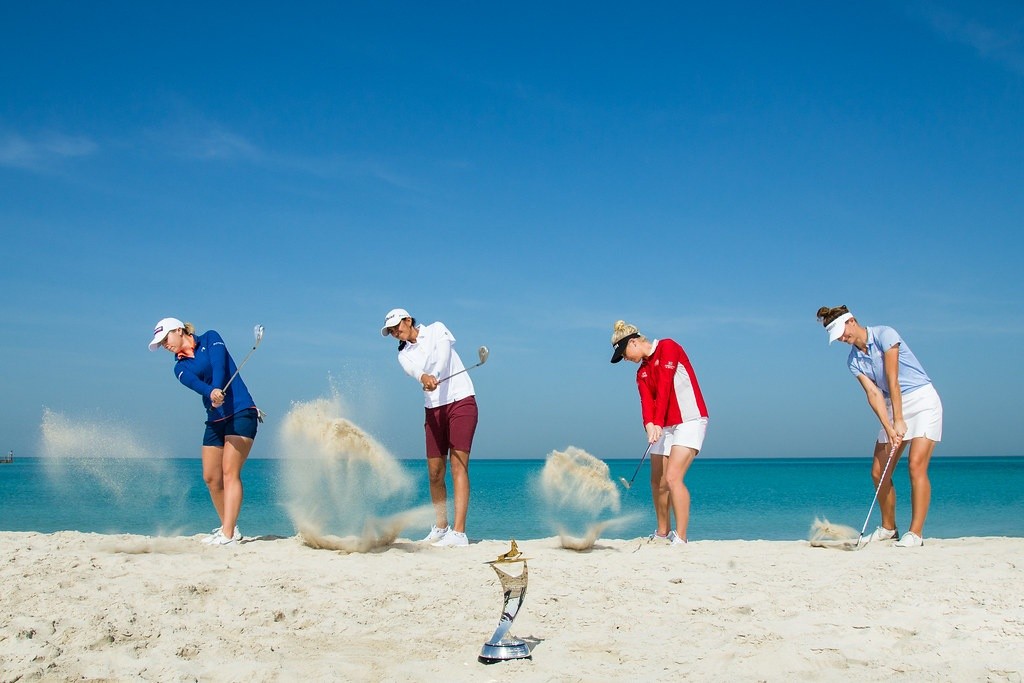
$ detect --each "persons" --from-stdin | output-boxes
[148,317,258,548]
[609,318,708,550]
[380,308,478,548]
[817,303,942,549]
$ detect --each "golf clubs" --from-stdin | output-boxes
[621,440,656,489]
[844,436,903,550]
[209,325,265,407]
[433,345,489,388]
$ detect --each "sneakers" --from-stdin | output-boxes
[668,530,689,553]
[200,525,242,549]
[431,531,469,548]
[895,531,923,547]
[640,530,672,545]
[416,524,452,546]
[862,526,899,543]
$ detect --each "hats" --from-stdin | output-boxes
[611,332,640,363]
[381,309,411,337]
[825,311,853,345]
[148,318,186,352]
